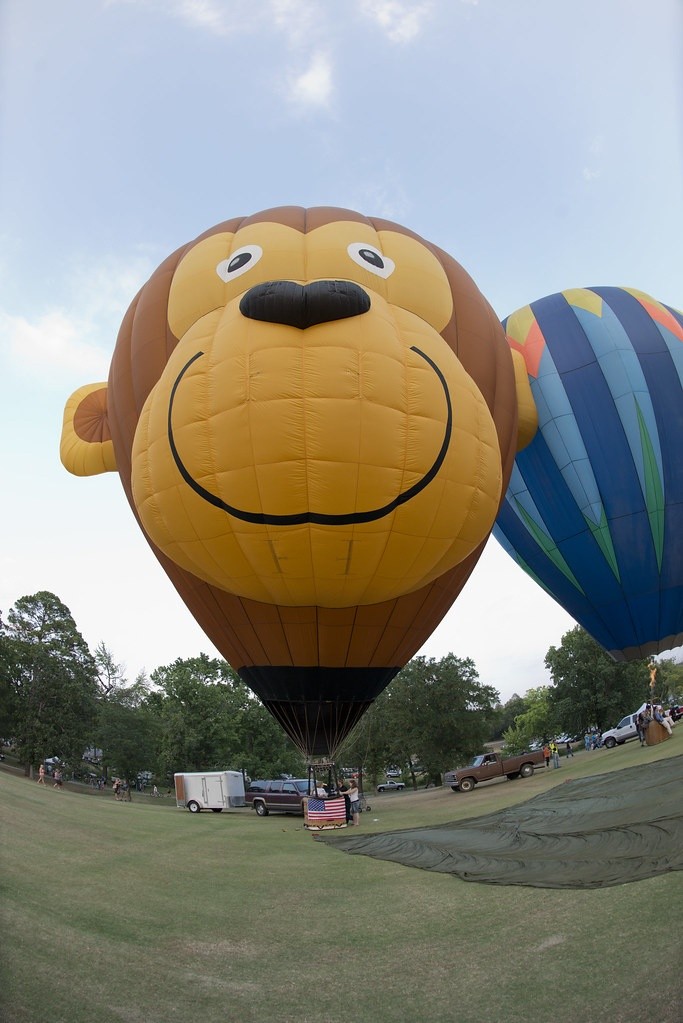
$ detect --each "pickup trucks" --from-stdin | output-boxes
[441,747,547,793]
[376,780,406,792]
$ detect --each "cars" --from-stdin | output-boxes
[601,708,664,748]
[528,725,601,752]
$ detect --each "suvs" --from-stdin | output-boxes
[245,778,327,817]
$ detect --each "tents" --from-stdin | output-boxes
[44,756,74,781]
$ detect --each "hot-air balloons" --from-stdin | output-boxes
[59,203,538,830]
[489,283,683,751]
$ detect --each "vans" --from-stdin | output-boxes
[339,767,366,779]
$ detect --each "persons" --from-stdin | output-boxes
[112,779,129,802]
[313,781,326,796]
[337,780,353,823]
[53,769,63,789]
[37,765,47,786]
[566,741,574,758]
[584,727,603,751]
[543,740,562,769]
[339,780,360,826]
[634,704,674,746]
[154,785,158,797]
[669,703,680,715]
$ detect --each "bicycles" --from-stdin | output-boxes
[150,791,164,798]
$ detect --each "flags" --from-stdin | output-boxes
[307,799,346,820]
